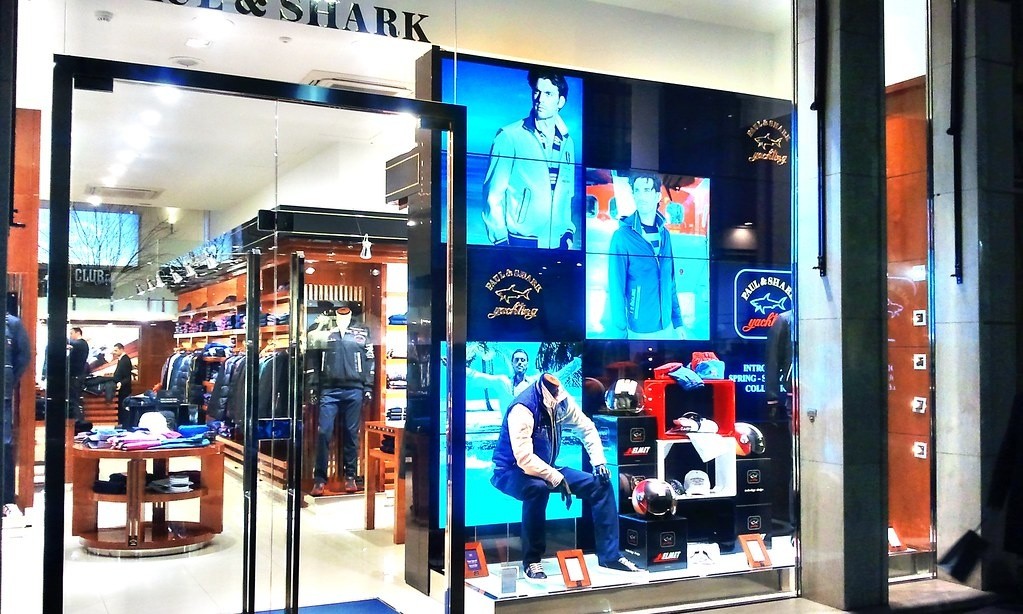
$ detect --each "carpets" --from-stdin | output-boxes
[233,595,402,614]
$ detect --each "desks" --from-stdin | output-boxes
[365,420,406,545]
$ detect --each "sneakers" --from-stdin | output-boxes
[523,562,547,583]
[345,479,358,492]
[312,482,326,495]
[599,557,643,576]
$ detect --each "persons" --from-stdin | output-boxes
[70,327,89,416]
[2,308,32,527]
[491,373,639,578]
[482,70,577,251]
[765,310,801,550]
[307,307,375,495]
[467,349,582,419]
[601,173,686,339]
[113,343,132,425]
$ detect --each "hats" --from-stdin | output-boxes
[138,412,170,435]
[684,470,711,495]
[181,303,191,312]
[196,302,207,310]
[218,296,236,306]
[666,479,686,496]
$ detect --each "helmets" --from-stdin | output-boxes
[734,422,766,459]
[632,478,677,520]
[605,379,646,416]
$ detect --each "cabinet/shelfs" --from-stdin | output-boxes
[172,239,407,497]
[71,440,225,557]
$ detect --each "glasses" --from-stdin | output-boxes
[514,358,525,363]
